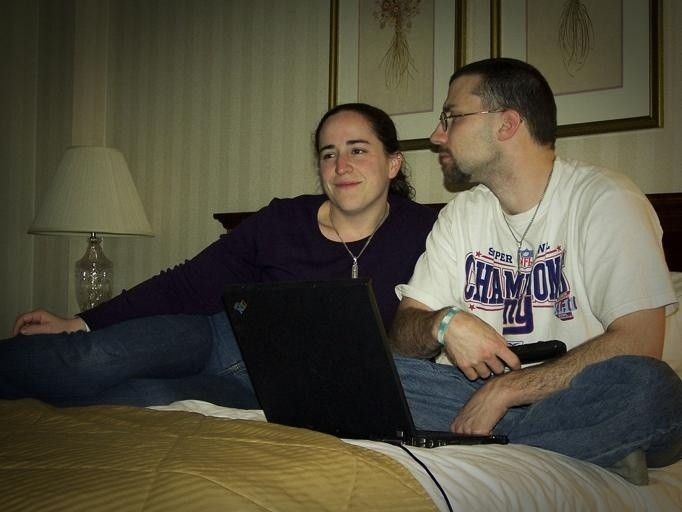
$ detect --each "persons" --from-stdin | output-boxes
[0,100,439,412]
[385,56,679,487]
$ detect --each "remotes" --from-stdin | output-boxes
[508,340,568,365]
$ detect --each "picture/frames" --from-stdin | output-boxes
[490,1,666,138]
[329,0,466,149]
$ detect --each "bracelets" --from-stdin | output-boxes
[431,307,463,347]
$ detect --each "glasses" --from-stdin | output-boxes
[439,107,523,133]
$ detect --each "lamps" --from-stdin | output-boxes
[29,148,156,314]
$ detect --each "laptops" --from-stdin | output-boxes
[221,278,509,448]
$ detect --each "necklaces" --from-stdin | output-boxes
[498,158,558,275]
[323,204,392,284]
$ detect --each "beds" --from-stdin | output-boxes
[1,193,682,512]
